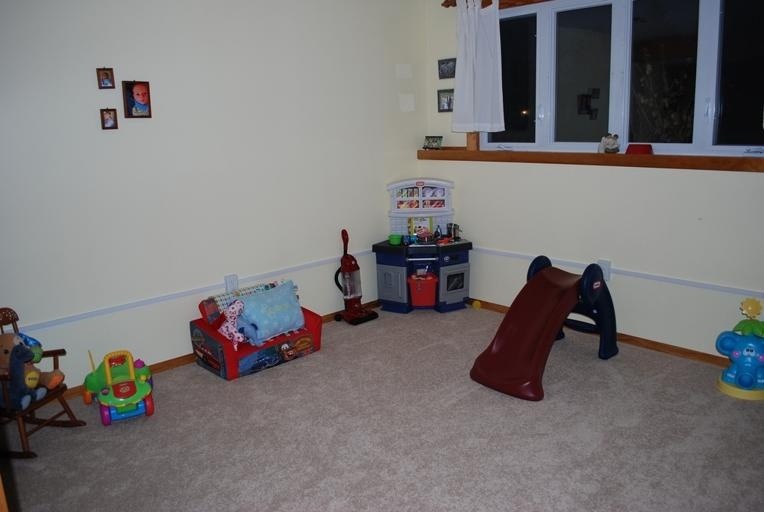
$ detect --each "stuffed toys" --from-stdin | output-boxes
[0,333,65,389]
[219,300,246,350]
[0,345,47,414]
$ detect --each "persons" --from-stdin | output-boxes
[440,95,453,107]
[446,62,454,73]
[440,62,447,73]
[104,112,115,126]
[604,132,619,153]
[131,83,149,117]
[101,72,111,85]
